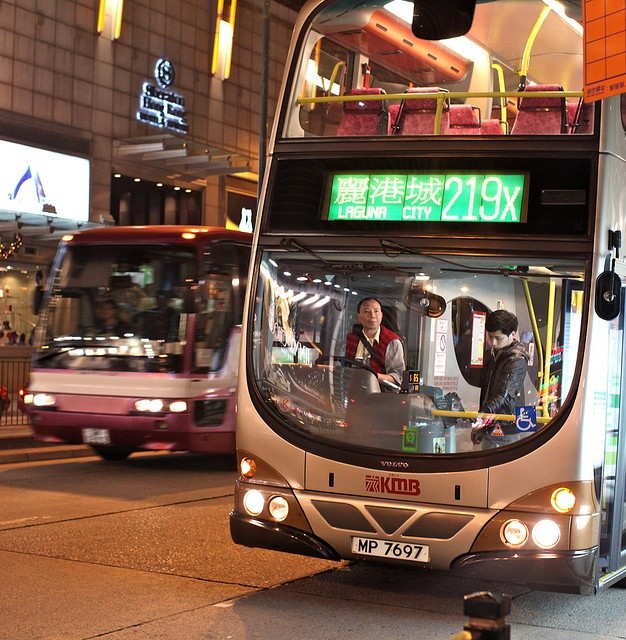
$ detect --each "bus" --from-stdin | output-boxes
[225,4,626,593]
[27,228,252,465]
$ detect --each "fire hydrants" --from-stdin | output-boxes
[449,590,517,640]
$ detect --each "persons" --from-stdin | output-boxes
[92,298,129,336]
[343,293,407,389]
[472,307,531,433]
[132,290,177,338]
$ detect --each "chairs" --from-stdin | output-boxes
[512,85,565,133]
[565,94,582,133]
[336,88,389,134]
[393,87,452,134]
[447,103,483,135]
[480,119,508,134]
[350,325,408,393]
